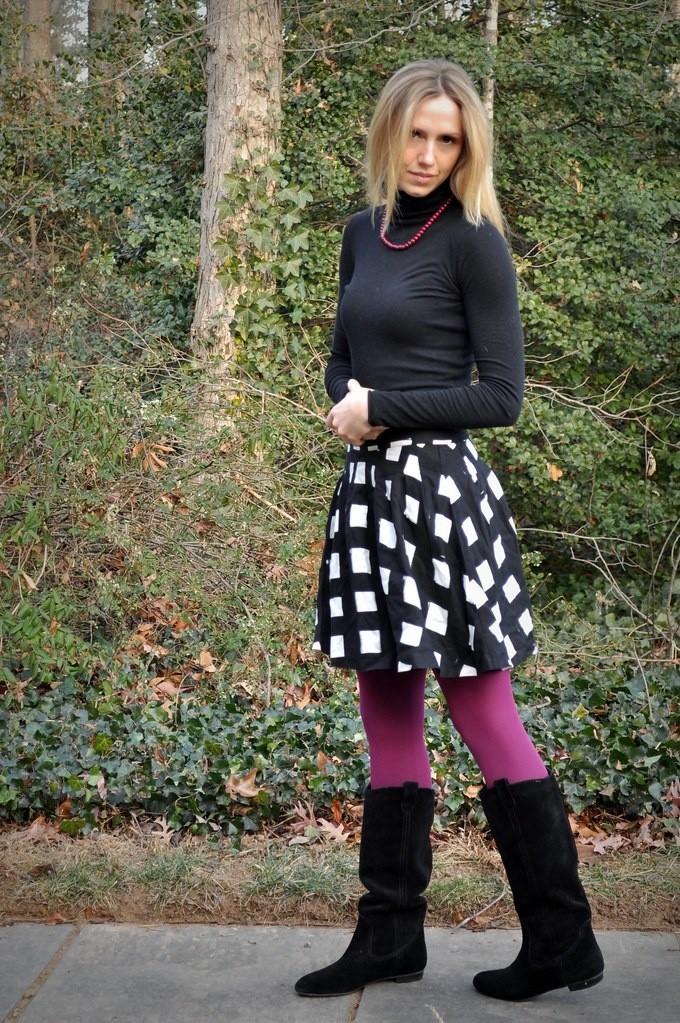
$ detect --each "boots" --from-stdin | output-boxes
[473,769,604,1001]
[294,781,435,997]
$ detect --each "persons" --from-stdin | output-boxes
[296,58,604,1002]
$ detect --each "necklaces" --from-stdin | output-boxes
[380,195,454,249]
